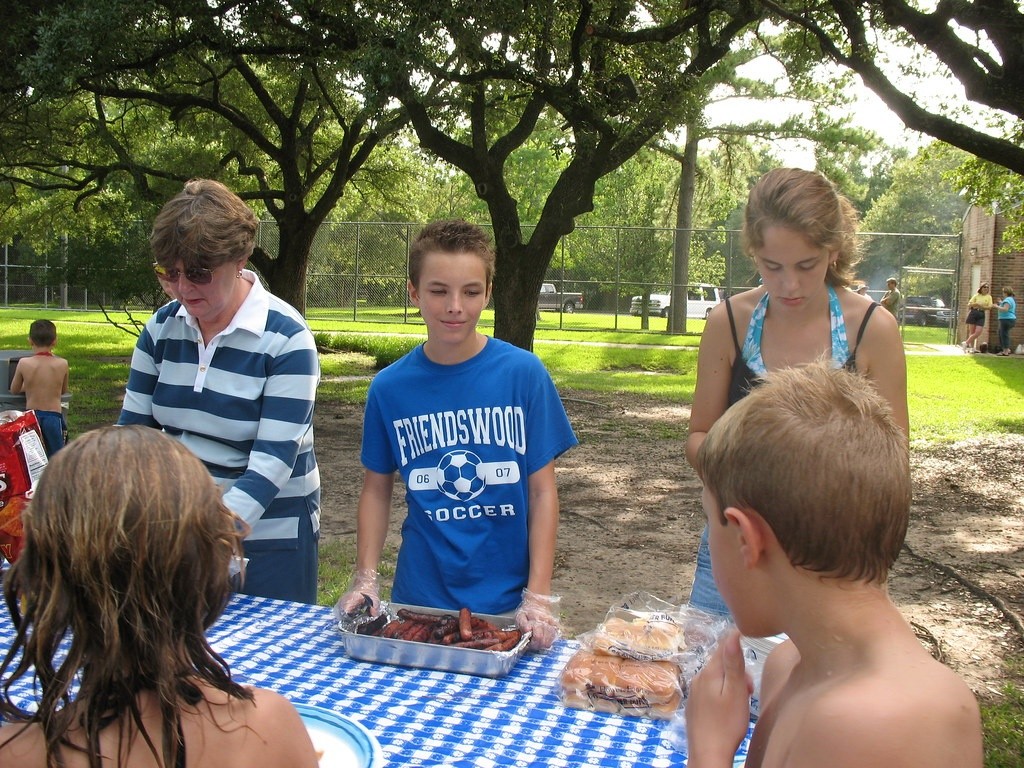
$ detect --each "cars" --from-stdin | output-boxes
[898,295,958,328]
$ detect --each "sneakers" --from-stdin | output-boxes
[968,347,974,353]
[962,342,968,354]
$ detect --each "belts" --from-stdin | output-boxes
[975,308,984,312]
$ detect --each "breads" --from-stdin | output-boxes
[559,619,683,719]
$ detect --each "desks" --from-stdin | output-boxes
[0,560,763,768]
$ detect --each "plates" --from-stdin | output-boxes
[291,703,383,768]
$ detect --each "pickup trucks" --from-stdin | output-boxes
[537,283,584,314]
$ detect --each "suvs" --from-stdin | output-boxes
[629,281,722,320]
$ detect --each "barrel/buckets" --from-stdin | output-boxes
[741,632,789,720]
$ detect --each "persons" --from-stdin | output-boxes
[10,318,68,459]
[992,286,1017,356]
[684,167,912,620]
[0,424,320,768]
[117,177,321,604]
[854,284,874,302]
[334,218,579,649]
[685,360,983,768]
[961,282,992,354]
[879,277,901,321]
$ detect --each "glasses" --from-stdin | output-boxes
[982,286,989,288]
[153,262,218,286]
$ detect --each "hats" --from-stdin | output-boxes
[856,284,869,292]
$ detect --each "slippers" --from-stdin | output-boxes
[997,351,1009,356]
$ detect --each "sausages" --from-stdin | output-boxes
[360,607,521,652]
